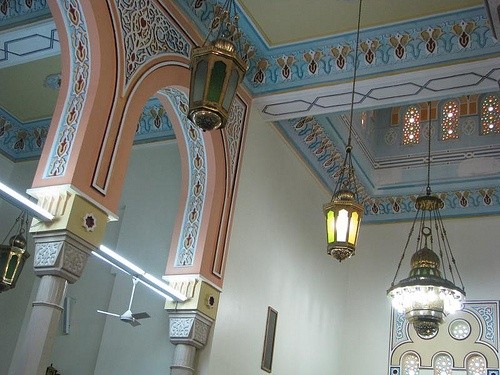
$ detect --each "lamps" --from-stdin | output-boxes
[91,245,187,302]
[0,181,54,225]
[187,32,246,133]
[321,177,365,264]
[385,227,466,337]
[0,228,30,293]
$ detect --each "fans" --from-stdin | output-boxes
[96,280,150,330]
